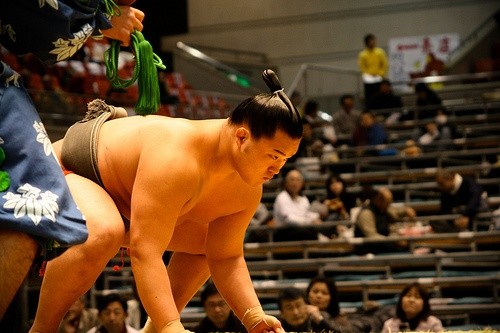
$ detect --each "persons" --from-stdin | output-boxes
[29,69,304,333]
[0,0,145,333]
[57,34,500,333]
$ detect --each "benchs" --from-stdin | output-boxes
[32,70,500,327]
